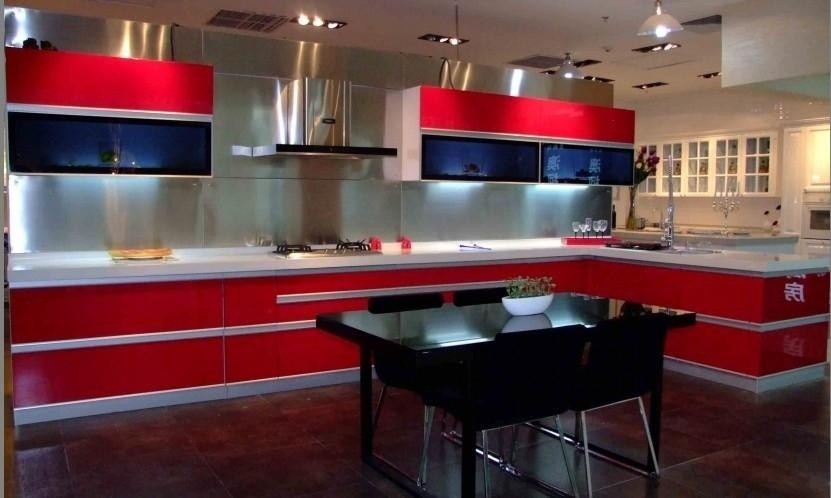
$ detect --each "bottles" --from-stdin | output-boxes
[613,204,617,229]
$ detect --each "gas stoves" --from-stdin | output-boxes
[273,241,384,260]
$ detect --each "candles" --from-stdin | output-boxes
[710,183,743,202]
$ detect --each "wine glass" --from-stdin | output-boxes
[572,218,609,238]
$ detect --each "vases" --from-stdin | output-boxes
[627,183,638,229]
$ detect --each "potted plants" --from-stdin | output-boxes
[503,273,558,316]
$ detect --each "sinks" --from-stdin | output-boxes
[605,242,670,251]
[661,247,722,256]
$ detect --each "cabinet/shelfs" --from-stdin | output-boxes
[802,114,829,204]
[658,136,713,197]
[780,126,802,234]
[713,132,775,196]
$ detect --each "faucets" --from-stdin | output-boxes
[661,155,674,247]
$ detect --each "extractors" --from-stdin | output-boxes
[251,73,400,162]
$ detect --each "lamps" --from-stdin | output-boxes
[637,0,686,41]
[555,51,586,81]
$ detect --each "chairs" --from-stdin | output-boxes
[316,293,697,497]
[422,321,582,497]
[503,309,670,498]
[451,286,580,469]
[365,293,502,495]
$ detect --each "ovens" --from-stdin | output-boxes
[802,193,831,240]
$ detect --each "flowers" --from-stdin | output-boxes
[630,145,661,219]
